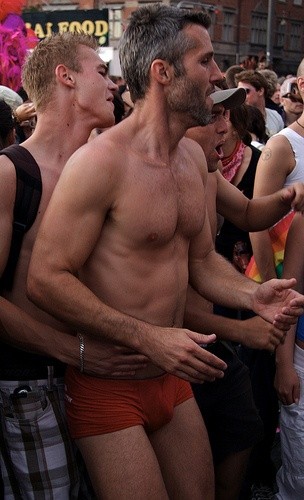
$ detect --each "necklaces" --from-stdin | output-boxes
[295,120,304,128]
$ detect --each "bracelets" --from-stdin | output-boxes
[77,334,85,374]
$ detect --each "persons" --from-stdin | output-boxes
[24,4,304,500]
[0,62,303,275]
[251,57,303,282]
[182,84,304,333]
[272,210,304,500]
[234,70,284,142]
[1,31,150,500]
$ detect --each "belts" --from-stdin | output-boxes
[0,361,67,381]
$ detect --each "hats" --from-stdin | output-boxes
[122,91,135,108]
[208,84,246,110]
[0,86,23,111]
[280,78,297,99]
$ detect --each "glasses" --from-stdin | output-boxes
[119,86,126,94]
[246,89,249,94]
[283,95,303,103]
[292,87,298,95]
